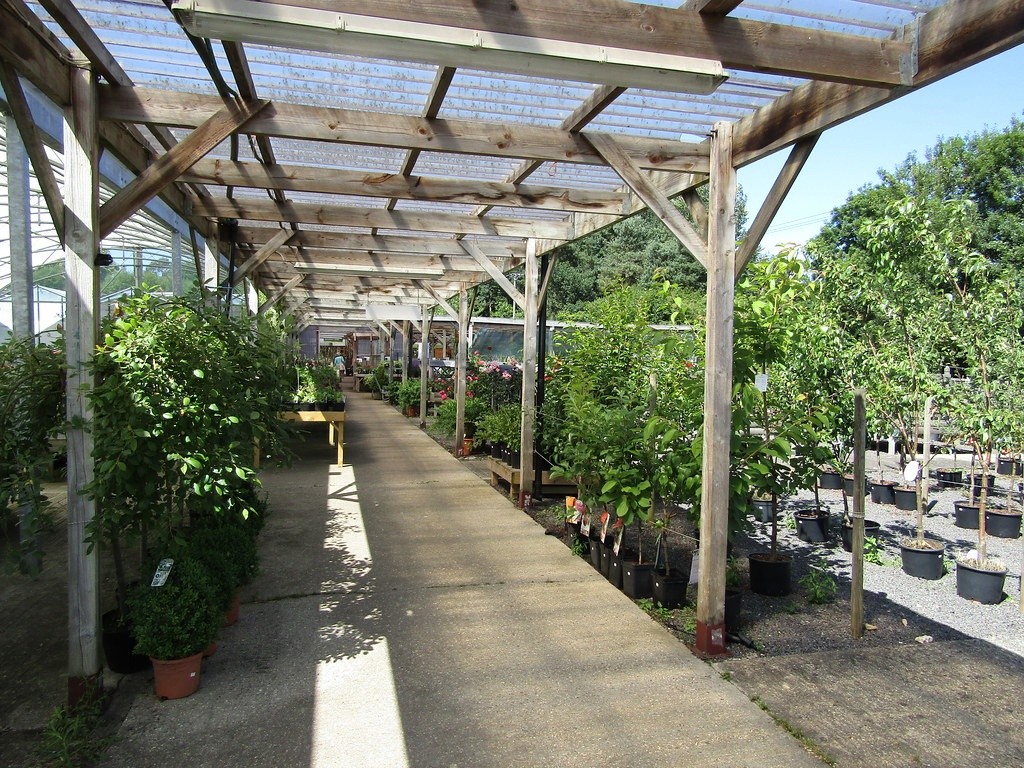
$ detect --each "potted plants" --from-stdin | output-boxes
[0,248,1023,698]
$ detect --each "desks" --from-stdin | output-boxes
[254,410,346,467]
[486,455,581,500]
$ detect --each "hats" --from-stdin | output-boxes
[337,353,341,355]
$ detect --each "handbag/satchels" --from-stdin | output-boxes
[340,365,345,370]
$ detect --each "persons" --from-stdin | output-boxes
[334,352,347,383]
